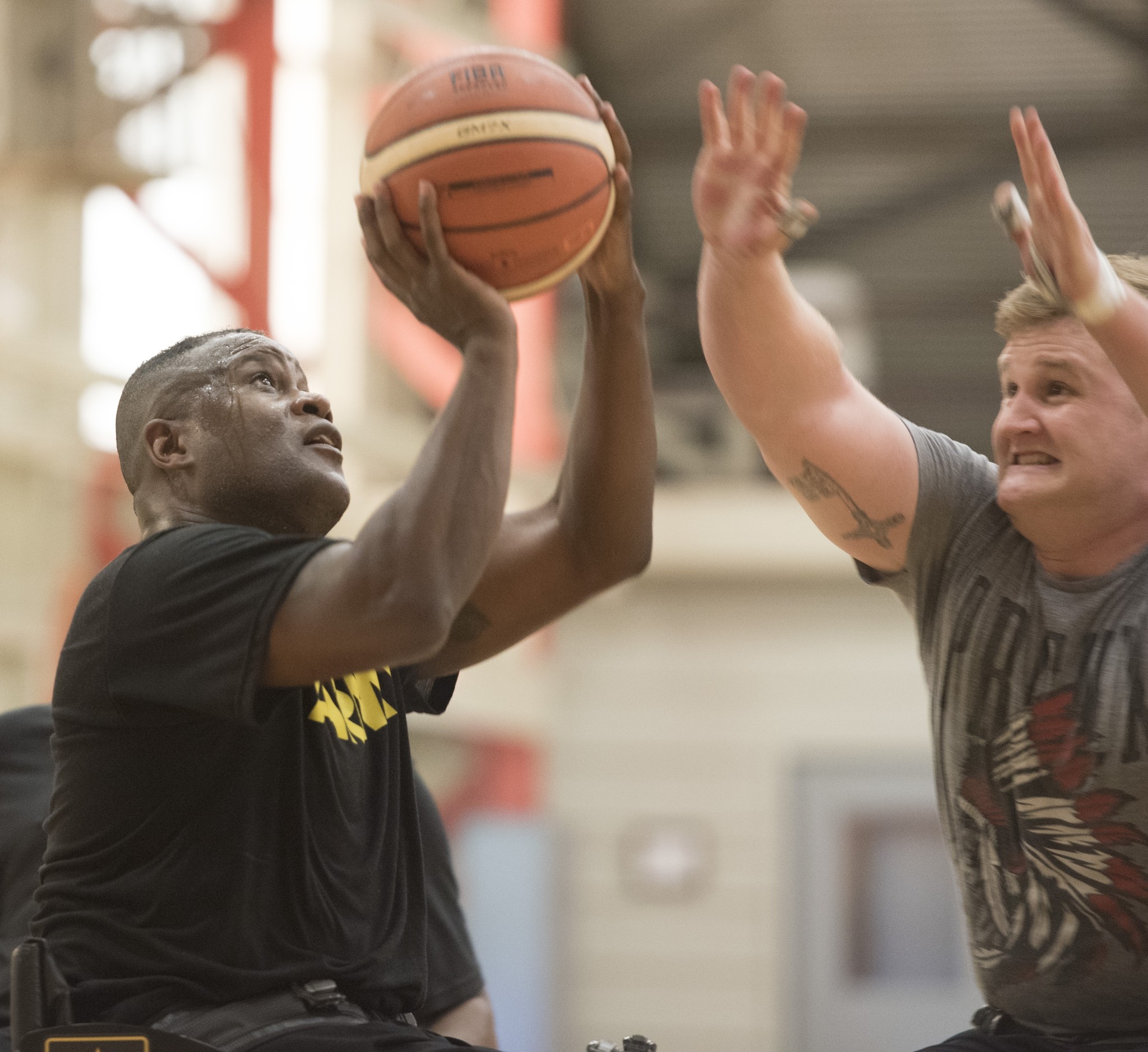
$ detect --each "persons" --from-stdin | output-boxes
[691,65,1148,1051]
[1,75,653,1052]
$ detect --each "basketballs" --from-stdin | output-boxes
[358,45,616,304]
